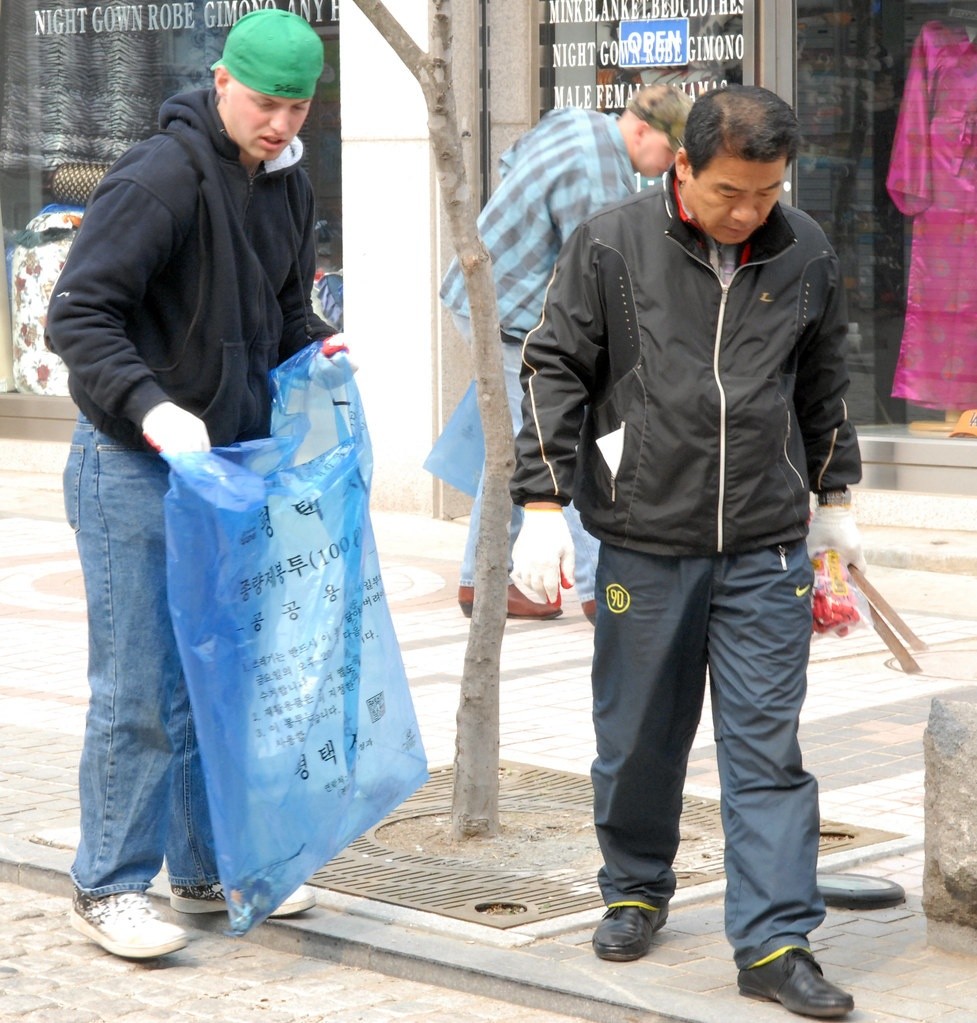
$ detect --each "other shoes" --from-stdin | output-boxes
[459,584,565,618]
[584,599,595,621]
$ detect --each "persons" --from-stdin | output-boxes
[439,82,696,625]
[44,9,361,958]
[510,84,863,1018]
[885,21,977,421]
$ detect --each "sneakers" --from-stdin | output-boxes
[171,881,316,914]
[69,888,188,958]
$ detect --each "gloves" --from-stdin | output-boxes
[510,501,575,607]
[806,504,872,577]
[812,551,860,637]
[142,400,211,472]
[310,334,360,389]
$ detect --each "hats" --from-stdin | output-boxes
[628,84,693,152]
[211,10,325,97]
[949,409,977,438]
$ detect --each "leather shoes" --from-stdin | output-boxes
[592,900,669,962]
[737,948,855,1016]
